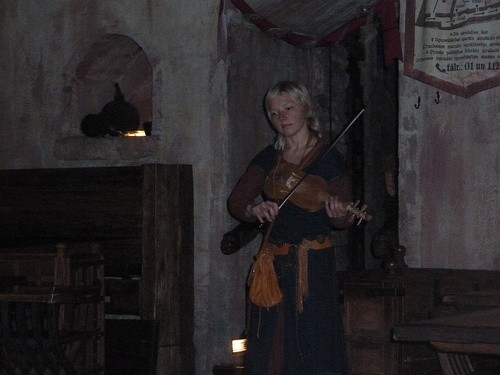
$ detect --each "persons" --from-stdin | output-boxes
[227,80,355,375]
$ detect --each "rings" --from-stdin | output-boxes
[262,205,265,209]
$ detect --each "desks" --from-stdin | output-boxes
[393,308,500,375]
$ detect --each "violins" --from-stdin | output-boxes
[262,163,372,225]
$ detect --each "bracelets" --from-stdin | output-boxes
[249,205,256,221]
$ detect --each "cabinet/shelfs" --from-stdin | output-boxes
[0,162,195,375]
